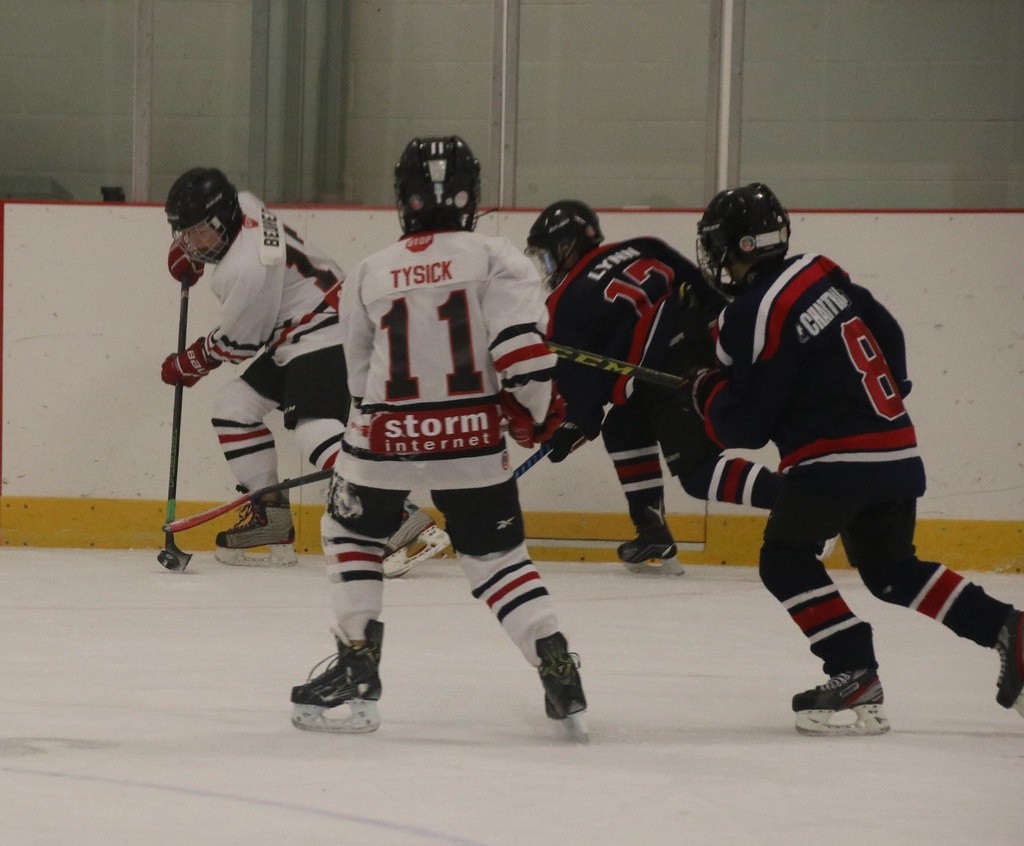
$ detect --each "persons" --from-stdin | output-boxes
[648,180,1024,739]
[518,197,838,575]
[286,135,593,746]
[156,166,452,582]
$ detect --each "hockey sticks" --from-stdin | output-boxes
[550,342,681,390]
[156,279,194,575]
[509,447,553,482]
[163,469,335,535]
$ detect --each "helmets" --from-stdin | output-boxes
[393,134,483,234]
[522,198,606,294]
[164,167,243,265]
[695,181,791,301]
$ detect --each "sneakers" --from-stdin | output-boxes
[615,523,685,577]
[791,665,891,736]
[815,529,840,562]
[214,477,300,567]
[995,604,1024,718]
[379,523,451,578]
[287,617,386,734]
[535,631,591,745]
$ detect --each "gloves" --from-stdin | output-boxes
[160,336,223,389]
[677,363,722,424]
[167,236,206,287]
[543,414,588,463]
